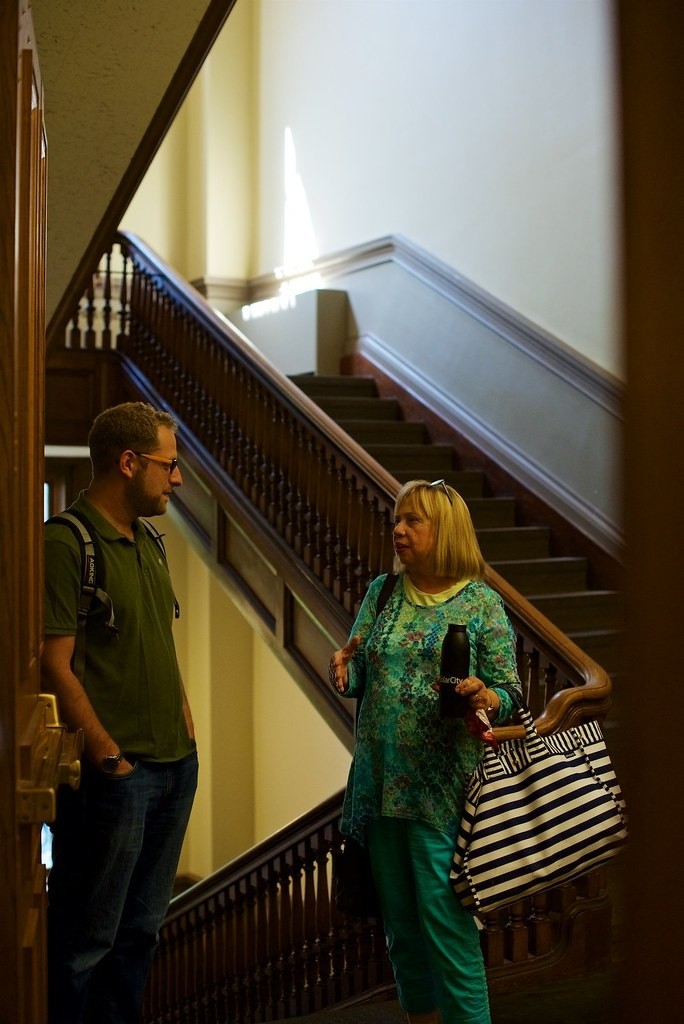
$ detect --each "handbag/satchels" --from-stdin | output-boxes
[449,683,630,915]
[334,845,382,917]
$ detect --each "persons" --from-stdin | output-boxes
[329,478,523,1024]
[42,401,198,1024]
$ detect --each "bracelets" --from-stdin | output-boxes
[485,693,494,712]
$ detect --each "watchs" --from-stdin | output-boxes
[101,751,124,774]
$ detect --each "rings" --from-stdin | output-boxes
[474,693,482,701]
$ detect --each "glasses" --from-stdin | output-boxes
[430,478,452,505]
[116,452,177,474]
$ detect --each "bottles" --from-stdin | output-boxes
[438,623,470,720]
